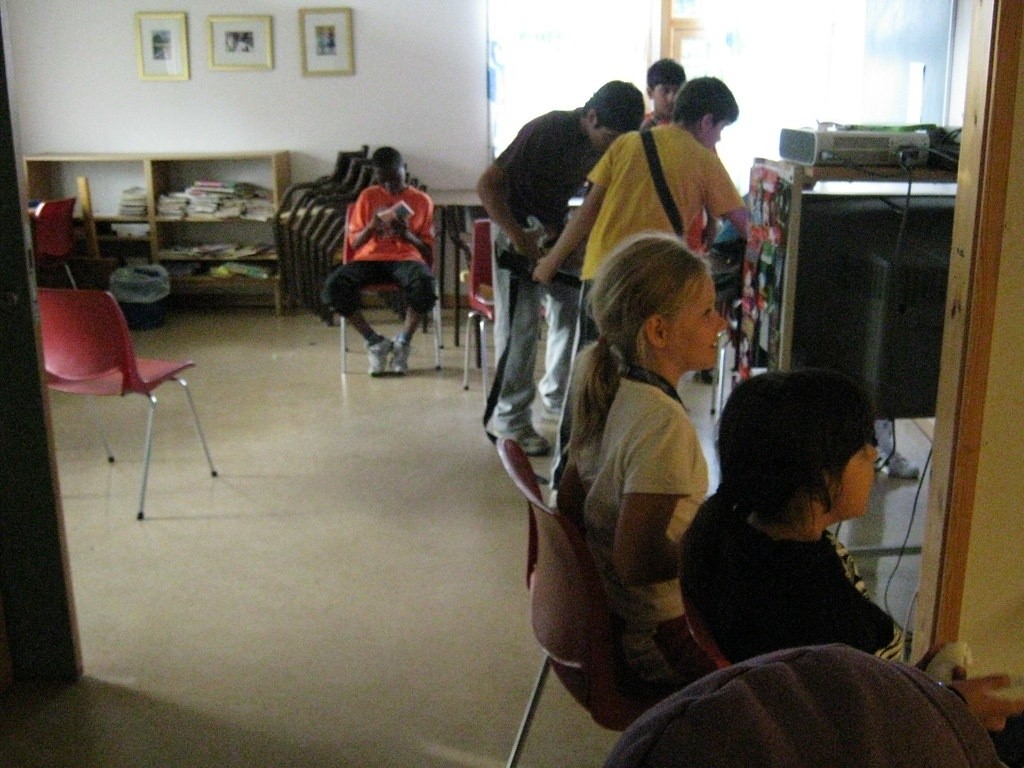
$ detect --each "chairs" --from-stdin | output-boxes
[339,207,728,768]
[32,197,78,290]
[38,288,219,523]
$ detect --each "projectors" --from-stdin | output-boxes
[779,128,929,168]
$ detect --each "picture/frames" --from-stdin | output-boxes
[206,15,273,72]
[134,11,190,82]
[299,7,355,77]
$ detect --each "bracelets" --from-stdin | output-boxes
[946,682,970,706]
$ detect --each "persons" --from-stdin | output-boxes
[677,368,1024,768]
[553,230,729,681]
[321,147,438,375]
[608,641,1012,768]
[479,54,765,459]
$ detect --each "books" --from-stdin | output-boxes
[155,177,271,223]
[172,239,276,261]
[227,262,272,280]
[117,186,147,218]
[376,201,415,227]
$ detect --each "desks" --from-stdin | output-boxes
[428,187,486,346]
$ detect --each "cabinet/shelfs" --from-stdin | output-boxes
[23,150,294,318]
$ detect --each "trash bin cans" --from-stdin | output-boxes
[116,266,168,331]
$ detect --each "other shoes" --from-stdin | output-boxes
[539,397,561,423]
[364,334,392,376]
[876,444,921,481]
[388,336,411,375]
[484,417,551,457]
